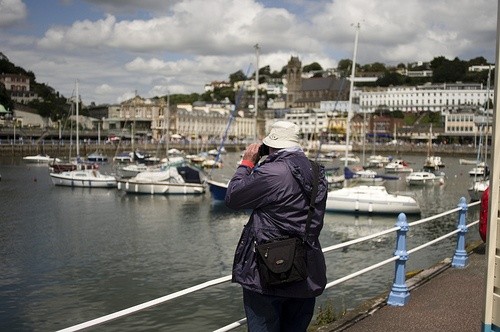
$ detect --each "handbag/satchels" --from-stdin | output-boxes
[255,239,309,288]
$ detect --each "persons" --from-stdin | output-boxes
[224,120,328,332]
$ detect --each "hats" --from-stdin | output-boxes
[263,121,303,152]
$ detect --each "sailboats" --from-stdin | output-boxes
[314,22,422,217]
[20,18,495,204]
